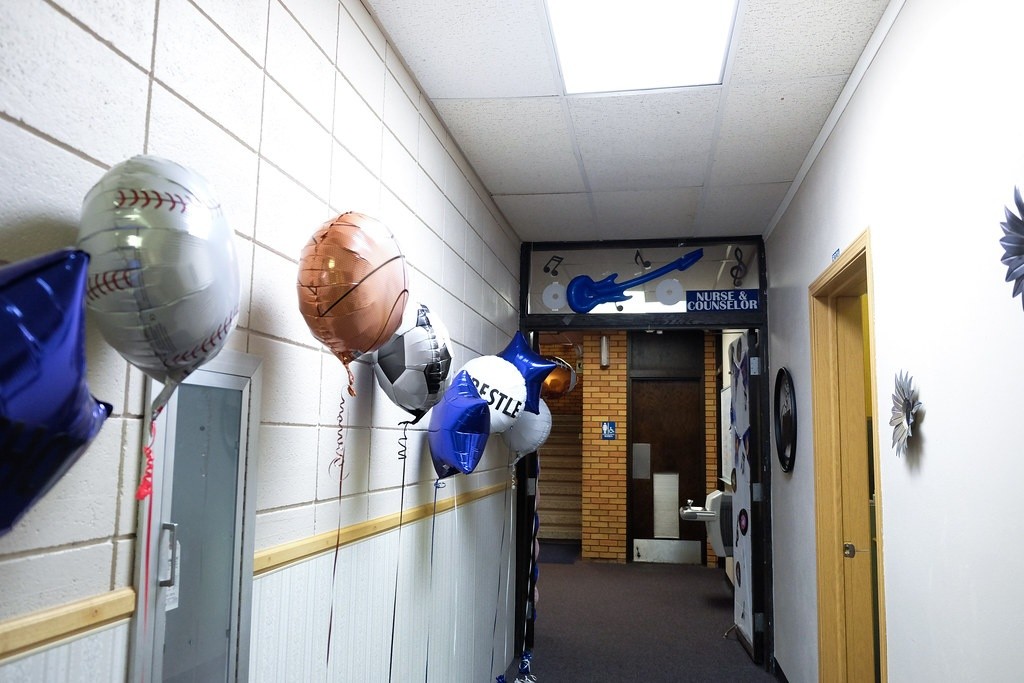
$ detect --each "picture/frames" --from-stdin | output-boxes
[774,367,797,473]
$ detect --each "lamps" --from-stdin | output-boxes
[599,333,611,368]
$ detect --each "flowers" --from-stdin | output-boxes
[999,185,1024,311]
[888,369,922,459]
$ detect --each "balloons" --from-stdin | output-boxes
[448,356,526,434]
[0,250,115,540]
[79,153,242,416]
[428,371,491,487]
[538,353,581,397]
[374,290,453,424]
[297,210,408,396]
[498,328,556,467]
